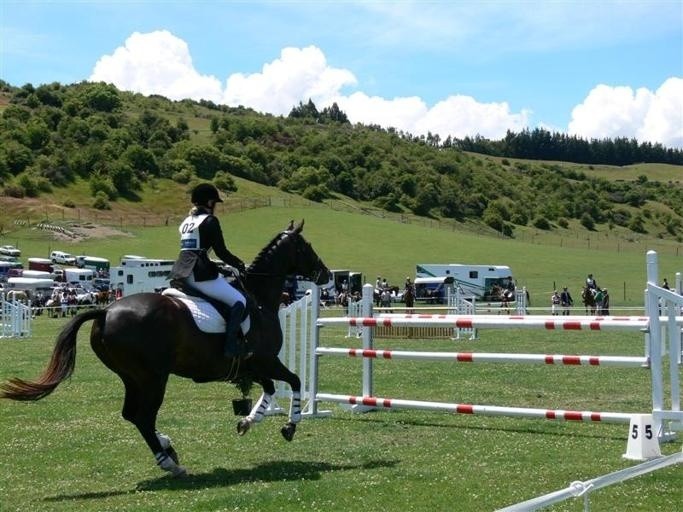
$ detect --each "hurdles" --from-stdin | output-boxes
[267,251,683,444]
[447,285,527,317]
[345,283,682,414]
[344,287,481,340]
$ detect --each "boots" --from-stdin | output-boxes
[222,300,255,361]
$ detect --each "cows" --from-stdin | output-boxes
[98,291,111,307]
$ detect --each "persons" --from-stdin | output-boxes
[336,274,416,318]
[603,288,609,314]
[164,183,258,359]
[662,282,668,289]
[24,283,124,320]
[661,278,666,285]
[593,286,605,314]
[585,273,597,290]
[497,292,509,314]
[551,291,561,315]
[560,284,573,316]
[503,275,515,296]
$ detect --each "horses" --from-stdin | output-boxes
[0,218,334,481]
[490,283,531,316]
[374,285,399,311]
[404,284,414,313]
[584,287,597,315]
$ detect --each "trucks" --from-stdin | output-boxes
[0,244,175,304]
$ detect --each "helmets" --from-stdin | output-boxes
[190,183,225,204]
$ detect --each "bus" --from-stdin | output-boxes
[285,268,406,305]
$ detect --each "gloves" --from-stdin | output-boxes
[235,261,246,275]
[219,267,233,279]
